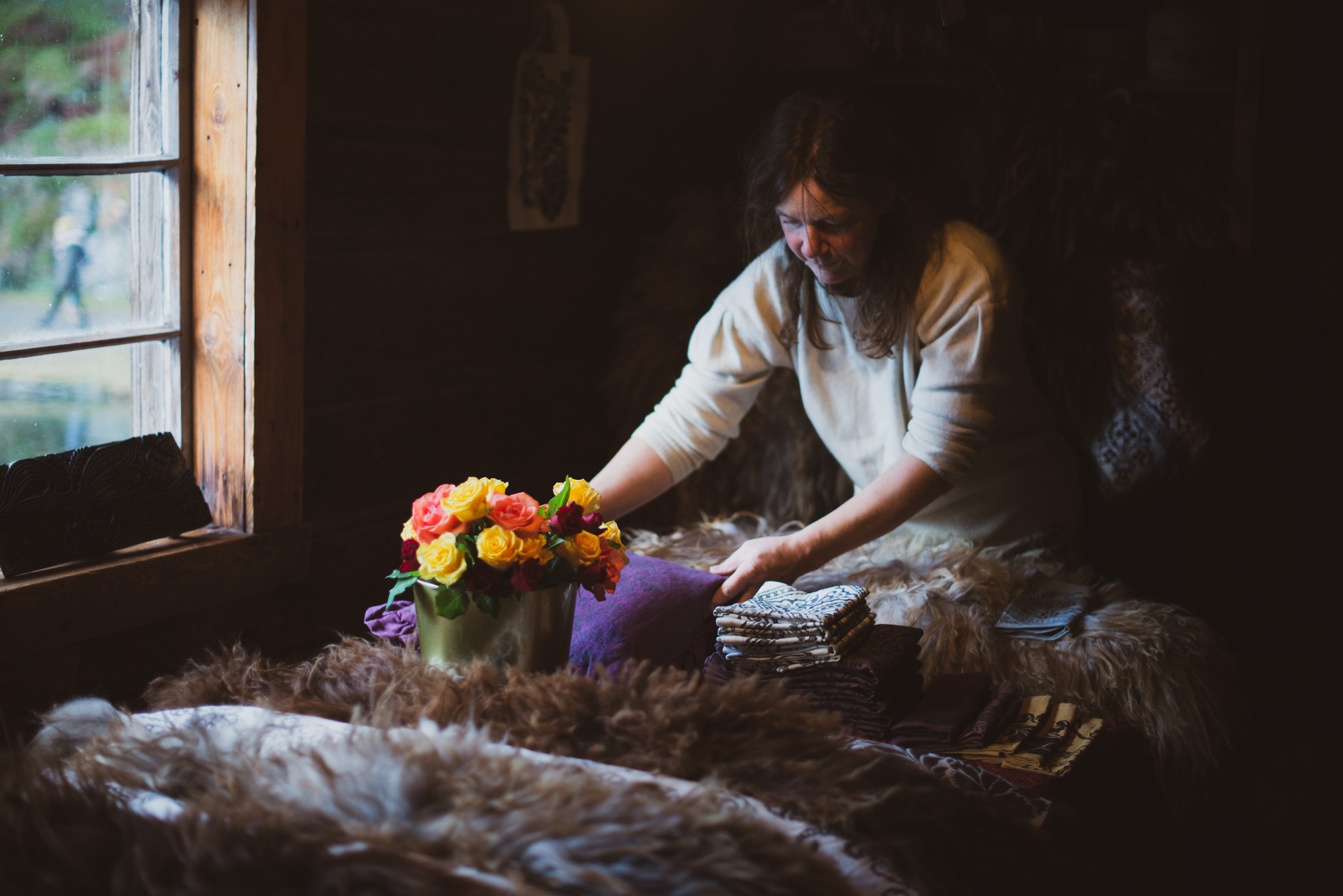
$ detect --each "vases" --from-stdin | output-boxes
[405,575,578,682]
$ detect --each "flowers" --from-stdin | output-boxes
[381,475,635,618]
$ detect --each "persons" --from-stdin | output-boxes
[585,95,1083,607]
